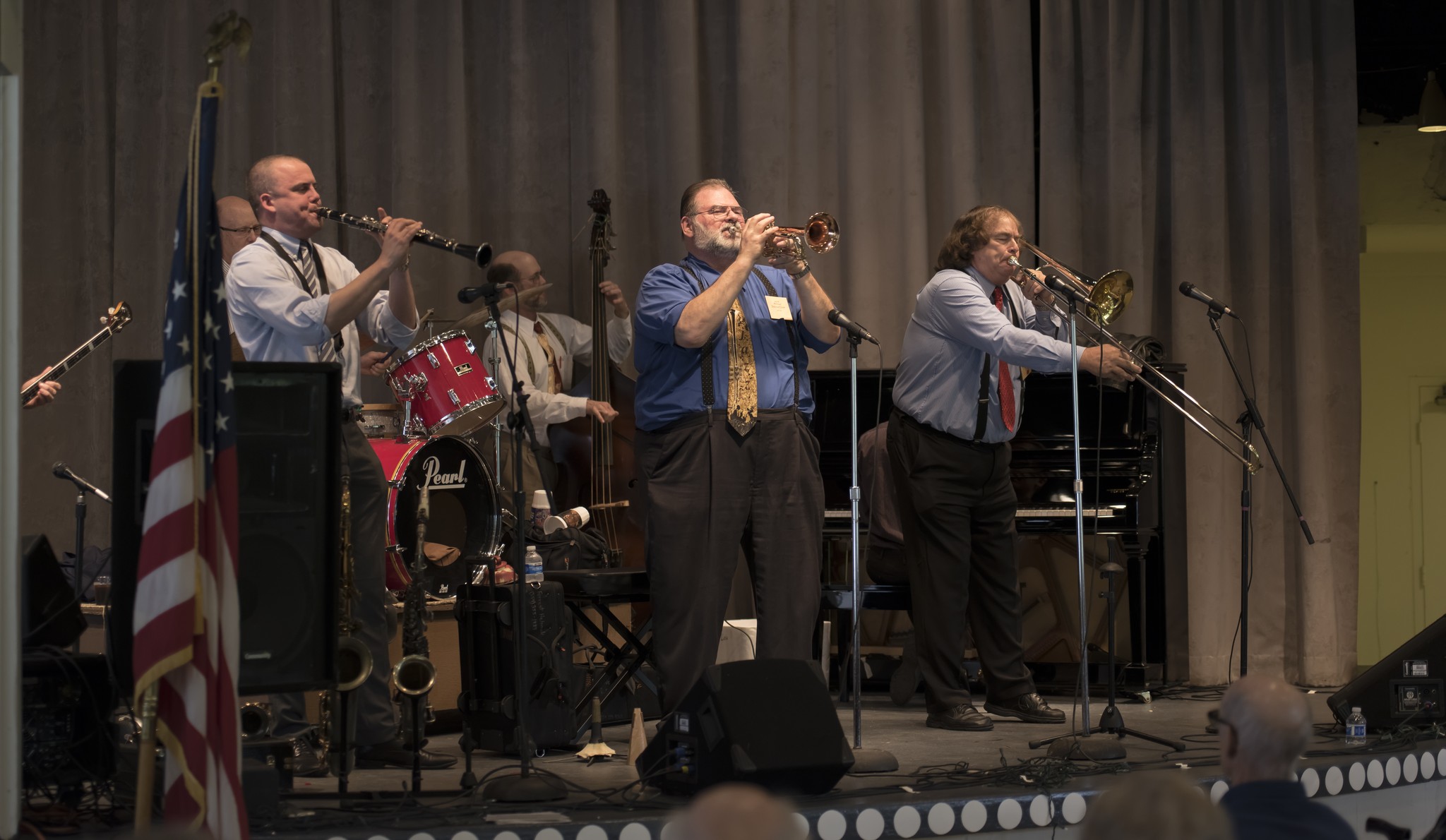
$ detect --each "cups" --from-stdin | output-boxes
[93,576,111,606]
[530,490,553,529]
[543,507,590,535]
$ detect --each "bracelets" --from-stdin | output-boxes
[392,253,411,272]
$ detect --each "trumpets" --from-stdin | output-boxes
[729,211,841,259]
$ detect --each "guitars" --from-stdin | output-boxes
[19,301,135,468]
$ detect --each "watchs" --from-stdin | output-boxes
[786,259,811,280]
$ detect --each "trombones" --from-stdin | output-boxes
[1003,237,1266,479]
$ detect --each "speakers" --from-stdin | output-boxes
[635,660,855,799]
[1326,614,1446,733]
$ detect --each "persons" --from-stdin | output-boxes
[215,197,393,377]
[676,787,799,840]
[1080,676,1358,840]
[883,203,1142,731]
[19,364,63,410]
[225,155,459,778]
[631,178,843,733]
[481,250,633,527]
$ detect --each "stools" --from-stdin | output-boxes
[549,567,657,746]
[821,583,914,699]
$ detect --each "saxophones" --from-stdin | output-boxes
[124,702,274,745]
[390,483,441,759]
[314,422,378,779]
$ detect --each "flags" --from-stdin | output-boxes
[135,86,244,840]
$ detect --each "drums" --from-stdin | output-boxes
[357,433,508,601]
[344,383,421,445]
[382,324,507,440]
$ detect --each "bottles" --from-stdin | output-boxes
[1346,707,1366,744]
[525,546,544,583]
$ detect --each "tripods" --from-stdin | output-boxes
[1028,537,1186,753]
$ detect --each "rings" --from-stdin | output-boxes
[784,239,789,250]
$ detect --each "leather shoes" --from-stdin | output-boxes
[282,738,331,777]
[983,691,1067,723]
[354,733,458,769]
[926,703,995,731]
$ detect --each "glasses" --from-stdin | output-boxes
[220,224,262,237]
[1207,709,1238,755]
[686,205,748,220]
[512,269,544,284]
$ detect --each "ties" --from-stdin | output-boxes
[533,322,564,395]
[299,240,338,363]
[727,297,758,436]
[993,288,1015,432]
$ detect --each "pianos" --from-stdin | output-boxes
[740,357,1193,708]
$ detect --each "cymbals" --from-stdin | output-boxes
[460,278,557,329]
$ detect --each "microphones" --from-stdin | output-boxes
[1179,281,1239,319]
[828,308,880,346]
[52,461,113,504]
[457,282,513,304]
[1044,274,1099,309]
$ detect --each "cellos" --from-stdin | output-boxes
[540,187,646,639]
